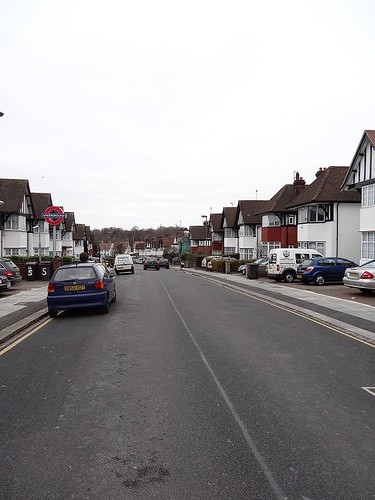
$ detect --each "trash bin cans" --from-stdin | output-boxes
[25,262,38,281]
[38,261,52,281]
[246,263,258,279]
[220,260,225,274]
[225,260,230,274]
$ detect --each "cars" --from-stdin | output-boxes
[158,258,169,269]
[297,257,360,286]
[342,259,375,293]
[47,262,116,318]
[238,257,268,276]
[134,257,145,264]
[0,258,23,292]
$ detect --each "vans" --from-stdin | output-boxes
[201,256,237,269]
[114,255,134,274]
[267,248,325,283]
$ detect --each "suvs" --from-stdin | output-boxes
[143,257,159,270]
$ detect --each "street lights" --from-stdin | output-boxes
[73,224,76,258]
[201,215,207,257]
[256,225,261,258]
[336,201,342,258]
